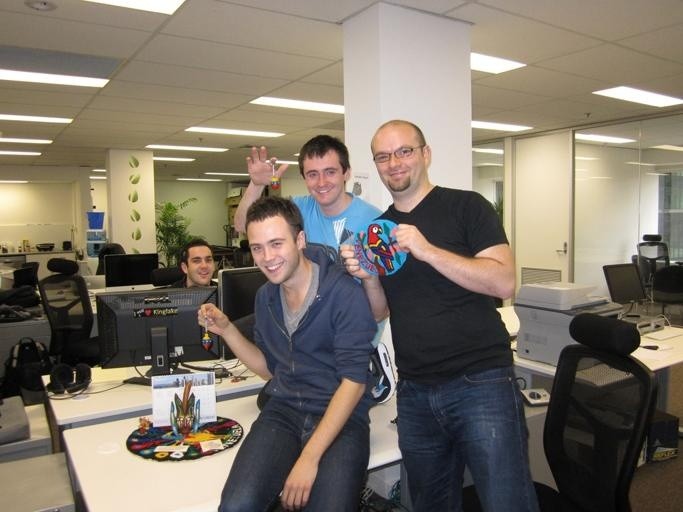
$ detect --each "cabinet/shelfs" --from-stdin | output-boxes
[0,251,76,282]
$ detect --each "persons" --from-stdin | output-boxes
[171,239,221,288]
[195,197,380,511]
[337,116,538,511]
[230,134,385,352]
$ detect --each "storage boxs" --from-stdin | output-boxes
[87,241,106,256]
[86,230,106,240]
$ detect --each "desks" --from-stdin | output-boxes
[503,317,682,475]
[0,283,170,378]
[63,375,549,511]
[41,358,270,451]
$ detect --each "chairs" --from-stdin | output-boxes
[39,275,109,365]
[151,267,183,287]
[47,257,78,275]
[14,268,35,289]
[653,266,683,318]
[632,234,670,315]
[456,314,658,512]
[22,262,38,275]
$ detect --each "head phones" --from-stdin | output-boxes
[46,361,91,395]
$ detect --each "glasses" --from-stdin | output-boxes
[372,143,428,164]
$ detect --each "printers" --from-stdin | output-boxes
[513,281,623,370]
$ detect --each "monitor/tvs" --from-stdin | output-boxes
[216,266,267,359]
[602,264,646,305]
[97,287,220,386]
[103,253,160,287]
